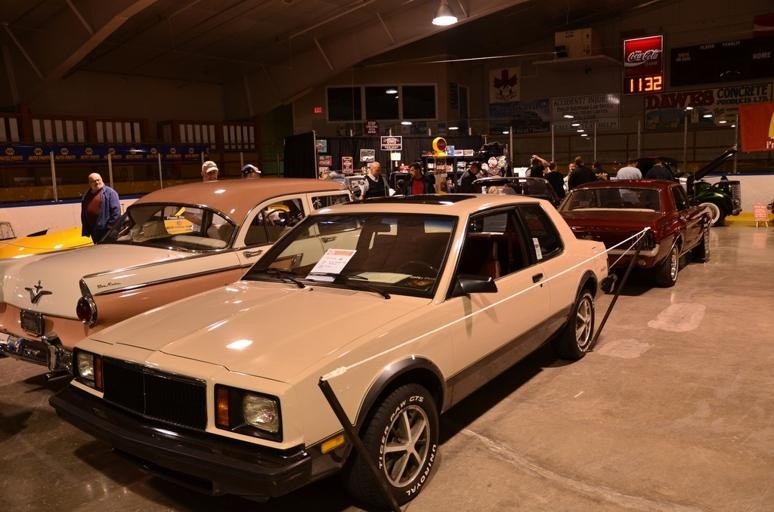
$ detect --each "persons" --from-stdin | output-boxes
[239,163,262,177]
[183,160,229,231]
[346,140,672,224]
[79,169,124,249]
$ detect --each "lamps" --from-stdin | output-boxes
[429,1,459,29]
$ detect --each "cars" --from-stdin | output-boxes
[468,176,562,206]
[628,145,741,227]
[1,204,289,265]
[1,176,396,377]
[47,192,611,505]
[525,177,713,287]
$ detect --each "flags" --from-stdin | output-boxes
[488,66,520,104]
[552,27,592,60]
[738,101,773,155]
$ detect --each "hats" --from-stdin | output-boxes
[202,161,218,174]
[242,164,261,174]
